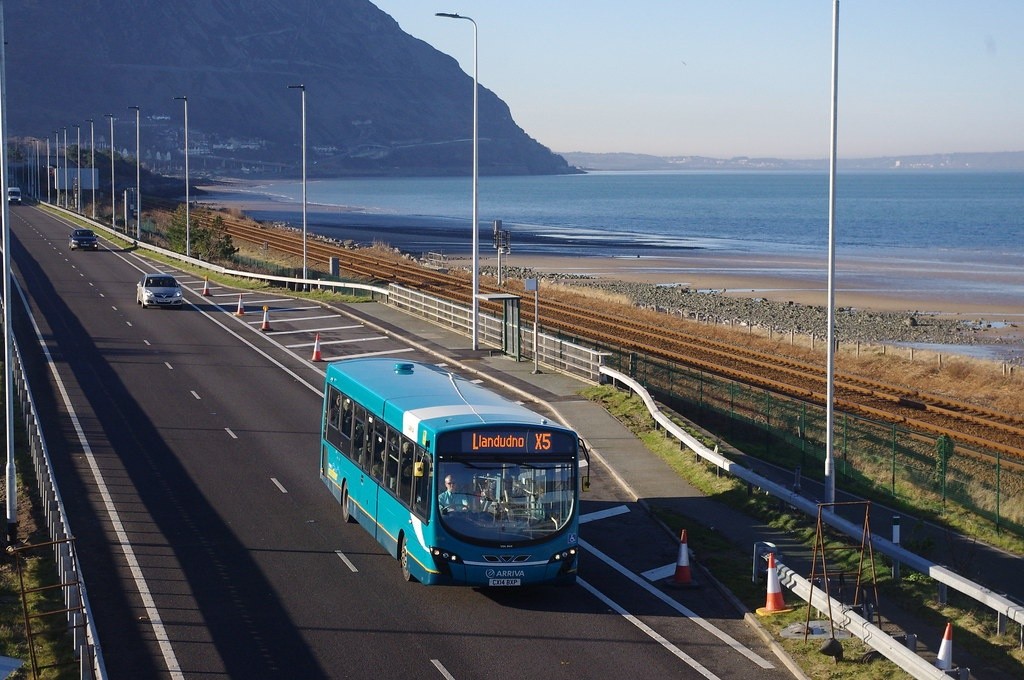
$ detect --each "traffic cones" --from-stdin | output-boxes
[236,294,247,315]
[309,332,325,363]
[669,530,696,586]
[259,305,274,332]
[755,552,795,614]
[932,623,958,677]
[201,276,213,297]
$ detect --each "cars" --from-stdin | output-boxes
[67,228,99,251]
[136,273,184,308]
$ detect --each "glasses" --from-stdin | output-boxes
[446,483,455,484]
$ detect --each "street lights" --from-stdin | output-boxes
[52,130,60,207]
[104,114,115,230]
[26,140,40,201]
[60,127,68,210]
[73,124,82,214]
[436,11,479,351]
[173,97,191,257]
[43,136,51,204]
[128,106,140,240]
[288,84,307,290]
[85,119,96,221]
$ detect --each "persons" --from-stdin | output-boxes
[436,475,471,515]
[324,393,428,507]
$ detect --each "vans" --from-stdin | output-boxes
[8,186,22,205]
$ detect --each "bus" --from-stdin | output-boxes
[318,355,592,586]
